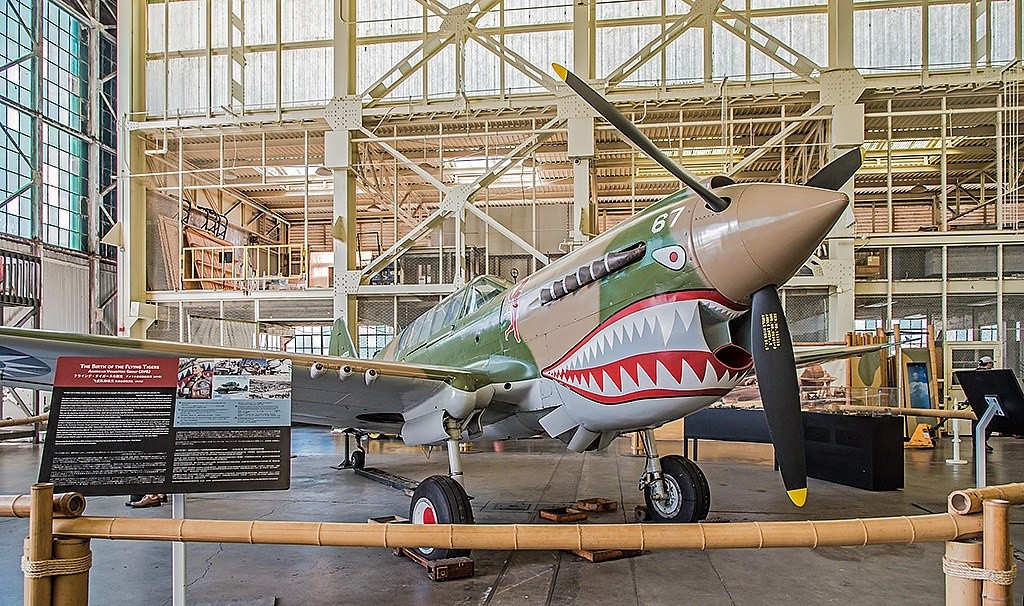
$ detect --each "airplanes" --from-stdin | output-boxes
[1,62,922,556]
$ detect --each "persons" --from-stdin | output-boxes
[972,355,997,452]
[177,361,212,399]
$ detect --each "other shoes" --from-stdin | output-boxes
[985,442,993,450]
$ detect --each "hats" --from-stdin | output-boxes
[978,356,996,363]
